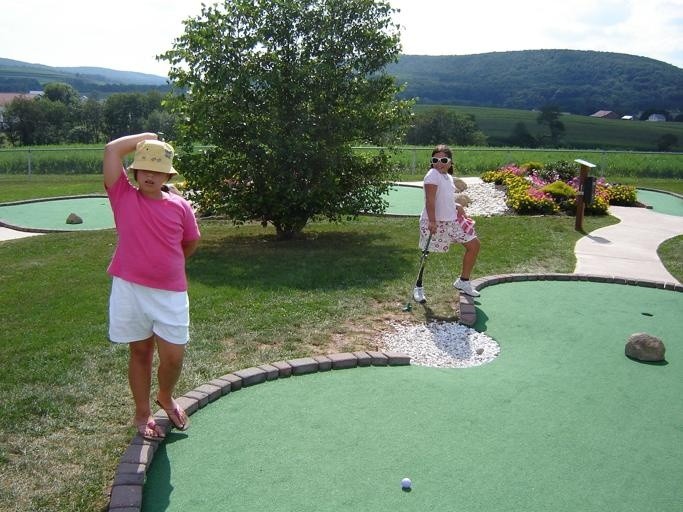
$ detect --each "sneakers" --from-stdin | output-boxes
[413,286,426,304]
[454,277,482,298]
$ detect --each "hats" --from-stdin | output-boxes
[127,139,180,176]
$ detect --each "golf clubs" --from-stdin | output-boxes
[402,234,432,312]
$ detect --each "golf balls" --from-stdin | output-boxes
[401,478,411,488]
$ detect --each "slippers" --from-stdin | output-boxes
[137,421,166,441]
[153,399,190,431]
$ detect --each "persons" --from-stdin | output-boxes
[412,145,481,302]
[101,132,201,442]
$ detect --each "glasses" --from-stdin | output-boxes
[432,156,452,165]
